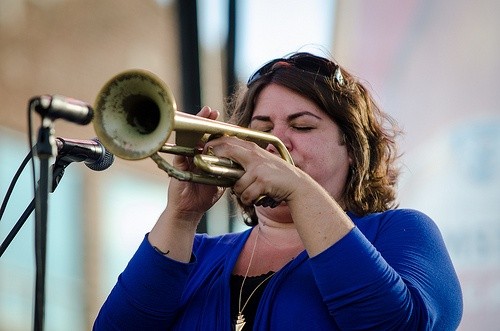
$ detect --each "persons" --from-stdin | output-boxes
[92,52,464,331]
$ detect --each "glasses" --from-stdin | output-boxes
[245,56,343,87]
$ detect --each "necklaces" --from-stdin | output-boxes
[233,225,298,330]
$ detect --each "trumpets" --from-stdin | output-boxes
[92,69,295,227]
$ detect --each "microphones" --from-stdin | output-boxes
[55,138,115,171]
[36,95,94,127]
